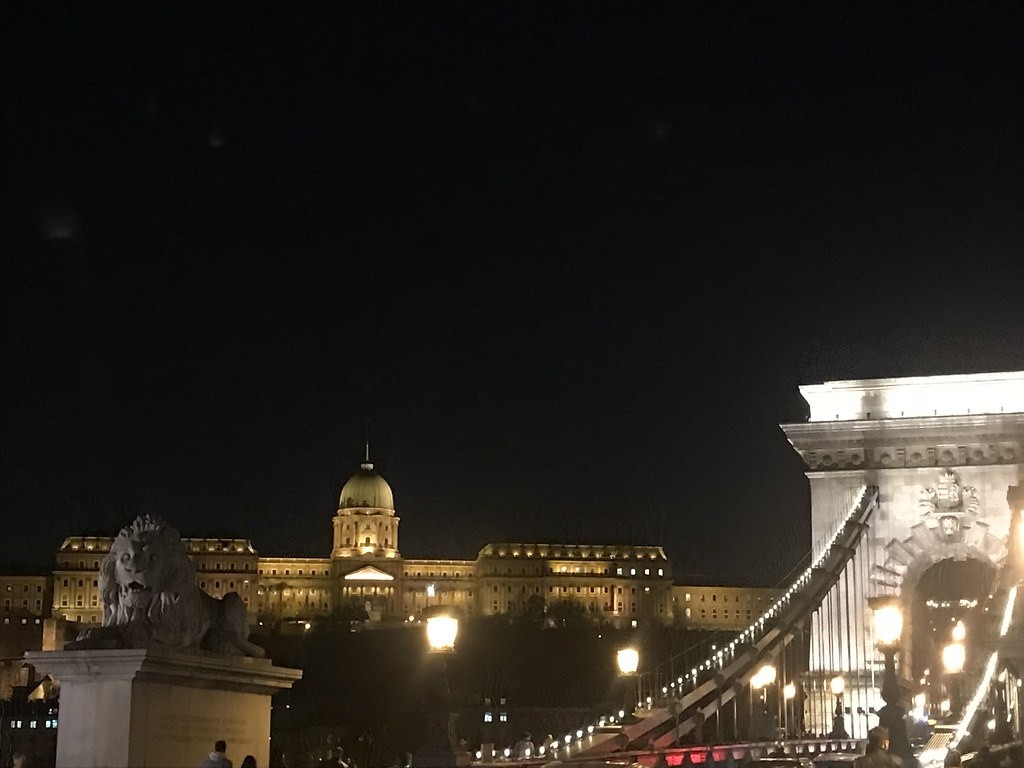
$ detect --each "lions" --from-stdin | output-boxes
[75,513,267,659]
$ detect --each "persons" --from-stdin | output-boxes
[241,755,258,768]
[944,749,964,768]
[515,732,535,759]
[394,751,412,768]
[201,740,232,768]
[543,734,555,754]
[453,737,474,768]
[321,747,349,768]
[853,726,904,767]
[968,746,1000,767]
